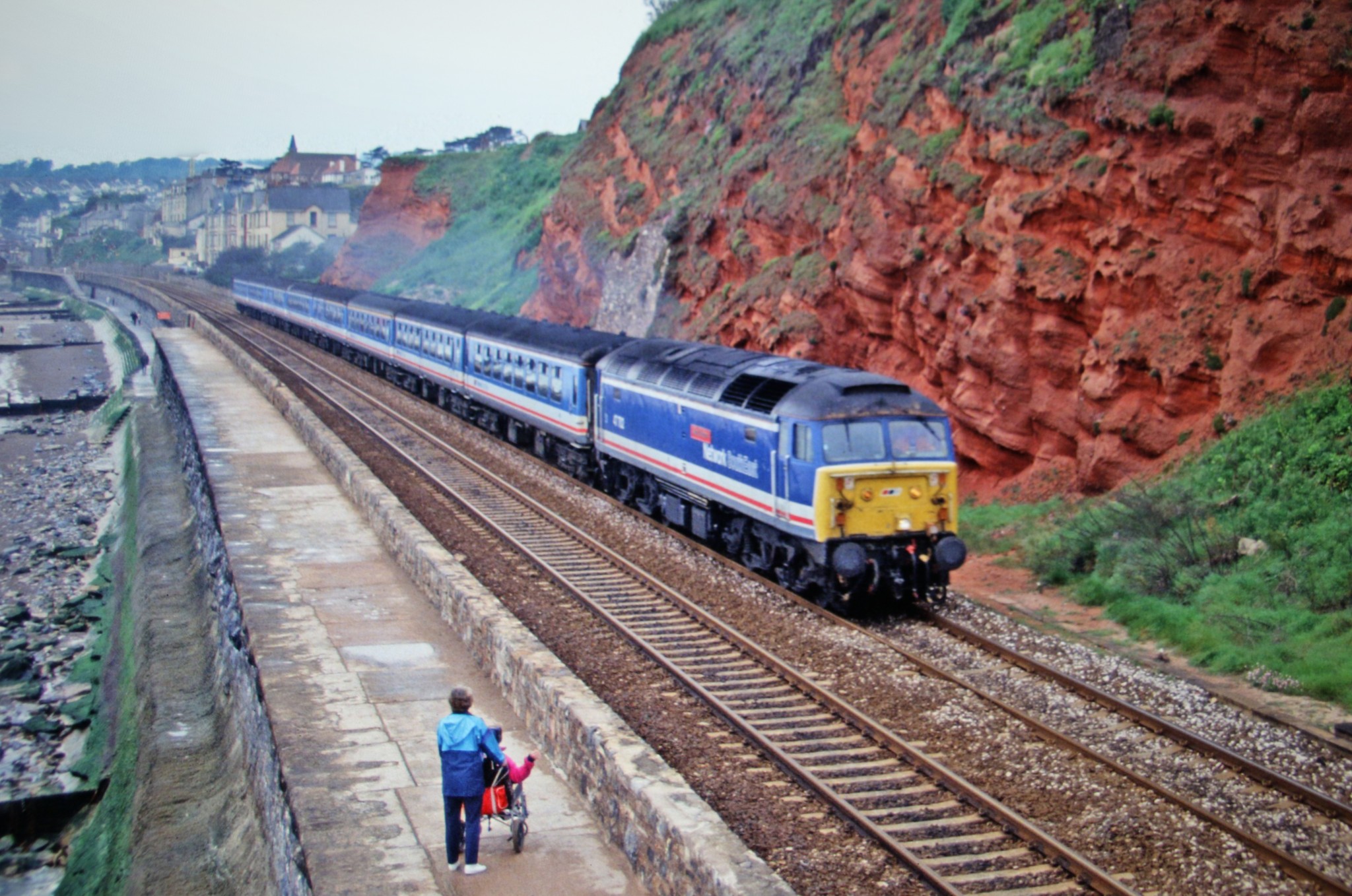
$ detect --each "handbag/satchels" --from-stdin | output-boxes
[480,784,508,816]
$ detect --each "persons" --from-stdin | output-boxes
[131,310,143,326]
[480,724,541,820]
[437,688,509,876]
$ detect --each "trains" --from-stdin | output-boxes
[231,267,968,628]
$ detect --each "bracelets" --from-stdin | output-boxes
[526,754,535,764]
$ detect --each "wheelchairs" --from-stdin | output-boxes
[459,761,529,854]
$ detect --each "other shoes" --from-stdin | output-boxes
[448,860,460,871]
[464,863,486,874]
